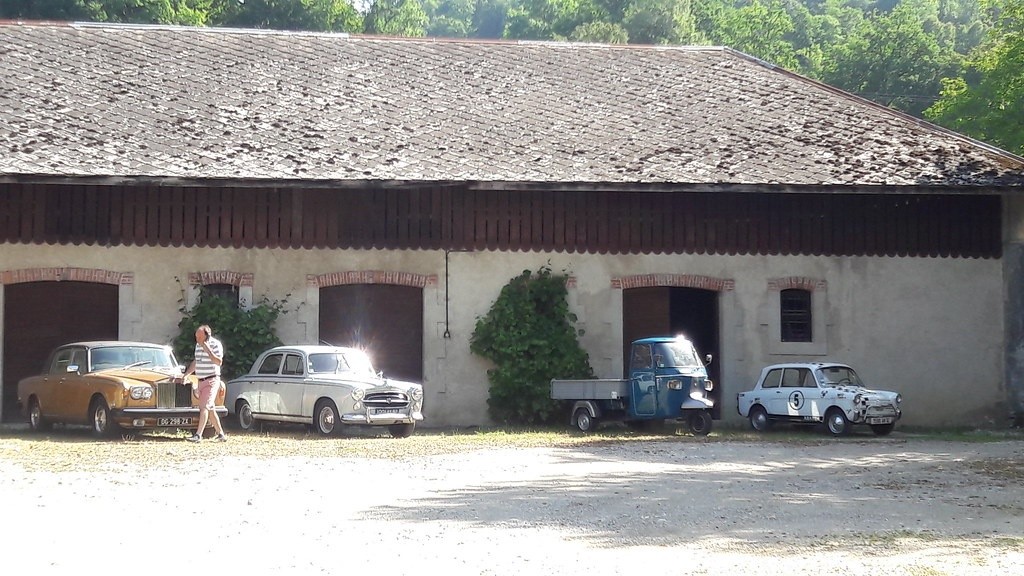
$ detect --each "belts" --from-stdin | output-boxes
[199,375,217,381]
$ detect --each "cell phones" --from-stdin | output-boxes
[206,334,210,342]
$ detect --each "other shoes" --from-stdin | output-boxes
[214,434,227,442]
[186,435,201,444]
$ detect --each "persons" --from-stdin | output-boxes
[179,324,228,443]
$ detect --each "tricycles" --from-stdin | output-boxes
[550,337,715,435]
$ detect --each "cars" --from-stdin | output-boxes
[17,339,228,439]
[736,361,903,435]
[228,345,423,438]
